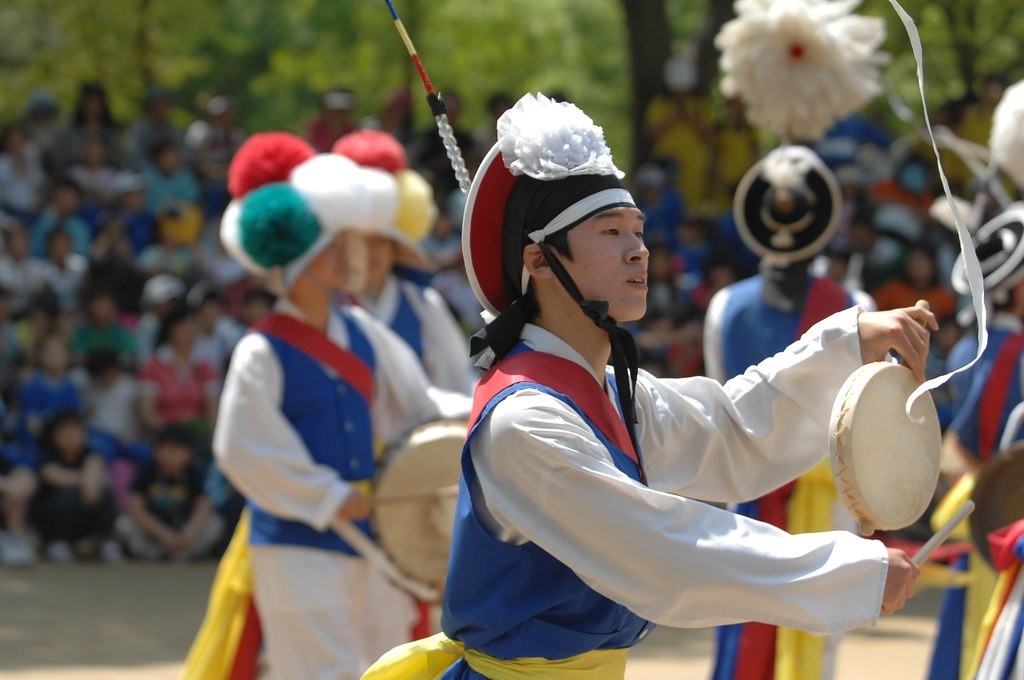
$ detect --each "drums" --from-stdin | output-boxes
[371,416,466,590]
[828,298,940,537]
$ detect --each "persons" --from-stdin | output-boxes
[0,0,1024,680]
[442,92,938,679]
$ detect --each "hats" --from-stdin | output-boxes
[731,146,842,264]
[144,273,186,306]
[218,126,372,297]
[333,128,439,259]
[949,81,1022,300]
[461,94,638,322]
